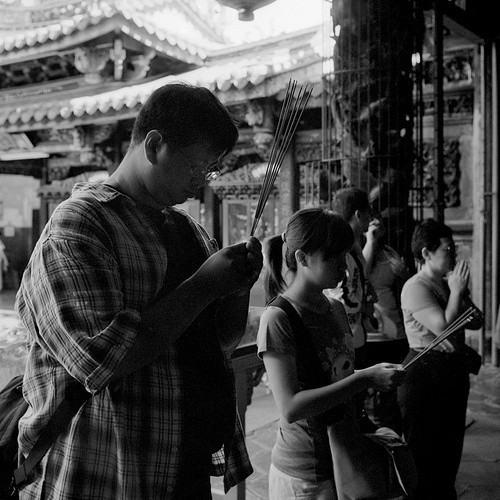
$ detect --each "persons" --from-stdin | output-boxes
[358,203,409,346]
[16,84,263,500]
[324,187,375,381]
[256,208,408,500]
[401,217,481,500]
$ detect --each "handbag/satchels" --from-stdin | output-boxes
[0,374,28,499]
[466,342,481,375]
[327,418,418,500]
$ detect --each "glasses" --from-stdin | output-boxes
[166,136,222,181]
[439,245,459,253]
